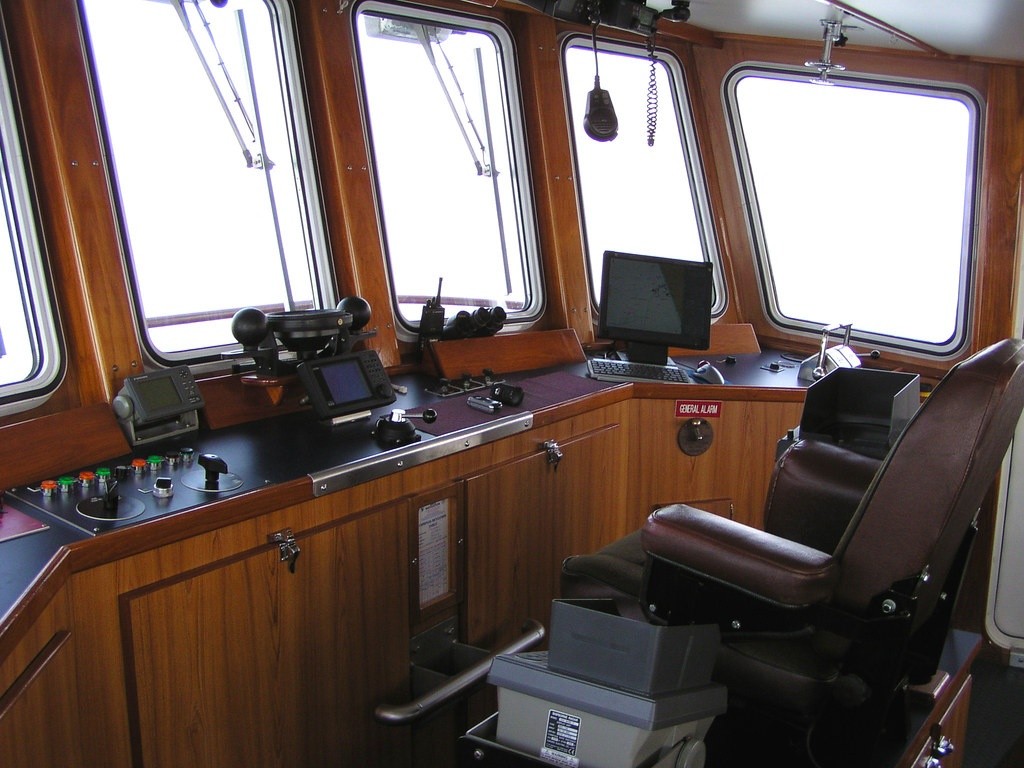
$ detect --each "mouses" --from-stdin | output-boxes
[693,365,725,384]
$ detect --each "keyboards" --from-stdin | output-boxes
[587,358,691,384]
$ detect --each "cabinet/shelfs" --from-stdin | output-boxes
[1,396,999,768]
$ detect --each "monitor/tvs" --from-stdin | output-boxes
[597,250,713,364]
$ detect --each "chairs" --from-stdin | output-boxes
[558,339,1024,748]
